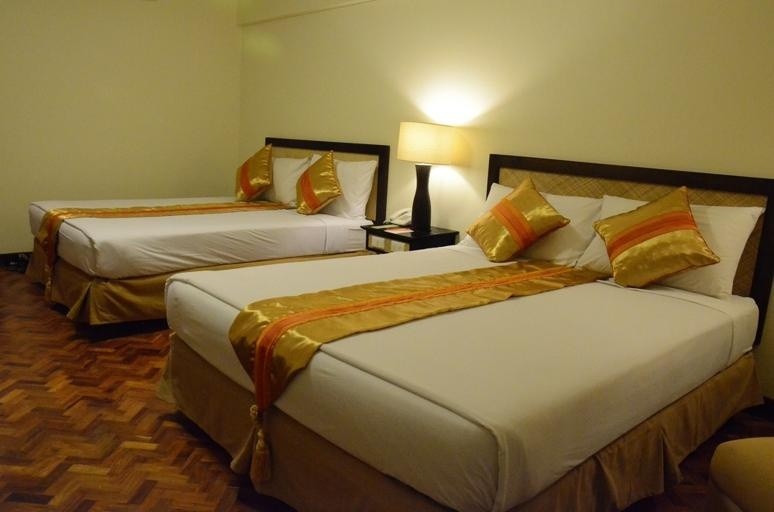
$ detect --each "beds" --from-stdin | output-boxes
[164,155,774,512]
[26,137,390,345]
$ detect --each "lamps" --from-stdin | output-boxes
[395,120,459,235]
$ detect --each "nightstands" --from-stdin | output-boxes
[360,221,459,255]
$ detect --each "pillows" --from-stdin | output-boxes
[575,193,765,301]
[236,143,272,202]
[465,176,569,264]
[296,150,341,215]
[295,152,377,221]
[592,185,719,287]
[459,182,603,269]
[260,157,308,208]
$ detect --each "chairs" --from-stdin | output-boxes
[704,435,774,511]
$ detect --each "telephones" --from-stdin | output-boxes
[389,208,412,227]
